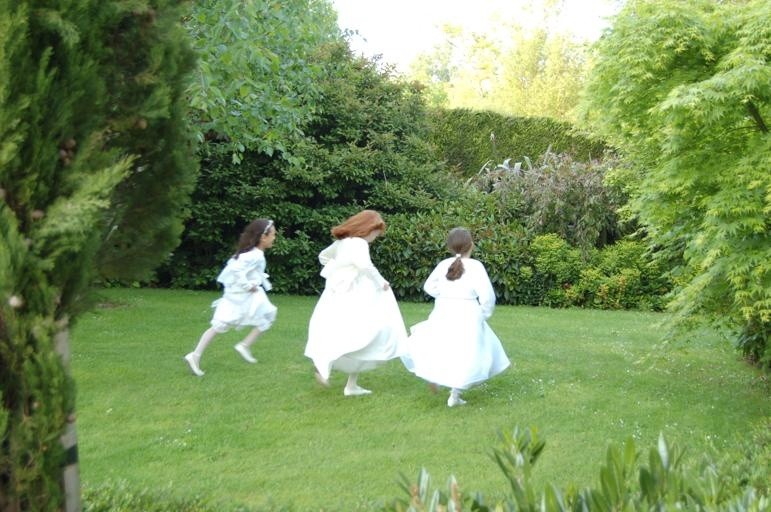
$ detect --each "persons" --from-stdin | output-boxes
[303,209,409,397]
[183,218,280,377]
[398,227,512,408]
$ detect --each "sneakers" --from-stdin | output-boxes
[344,384,372,396]
[184,351,205,376]
[447,397,467,407]
[233,341,258,364]
